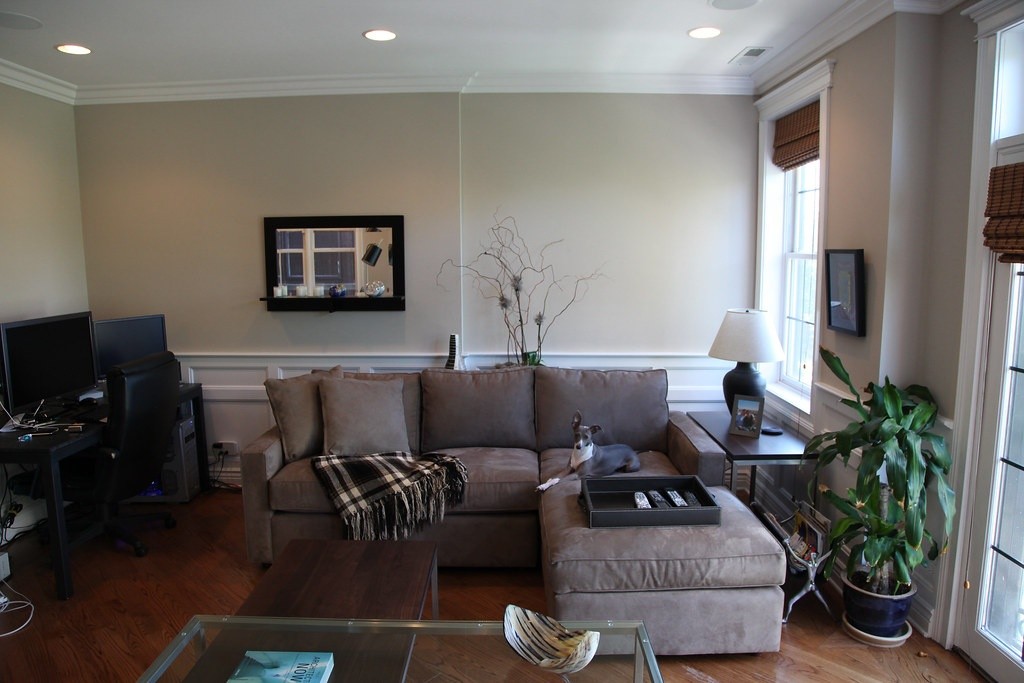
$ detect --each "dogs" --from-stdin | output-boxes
[534,410,640,495]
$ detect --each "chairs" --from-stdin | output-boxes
[68,351,181,555]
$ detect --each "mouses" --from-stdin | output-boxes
[78,397,98,406]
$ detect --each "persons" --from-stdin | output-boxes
[735,408,756,432]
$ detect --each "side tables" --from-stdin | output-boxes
[687,411,821,513]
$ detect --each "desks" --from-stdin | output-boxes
[0,381,211,602]
[179,536,440,683]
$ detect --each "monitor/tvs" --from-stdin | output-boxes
[0,310,99,429]
[91,314,168,383]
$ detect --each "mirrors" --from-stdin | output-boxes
[260,216,407,313]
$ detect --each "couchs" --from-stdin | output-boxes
[239,368,786,655]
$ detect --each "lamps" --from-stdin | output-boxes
[353,228,394,266]
[708,307,786,417]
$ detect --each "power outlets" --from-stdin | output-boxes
[217,441,238,456]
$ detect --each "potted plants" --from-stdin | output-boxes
[798,346,956,637]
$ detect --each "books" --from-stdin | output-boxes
[789,500,831,567]
[226,651,334,683]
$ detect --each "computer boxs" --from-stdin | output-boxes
[154,415,201,501]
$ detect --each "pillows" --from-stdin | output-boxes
[318,374,411,458]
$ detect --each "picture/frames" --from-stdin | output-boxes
[825,250,865,335]
[727,394,765,438]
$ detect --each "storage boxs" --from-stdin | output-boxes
[579,471,722,526]
